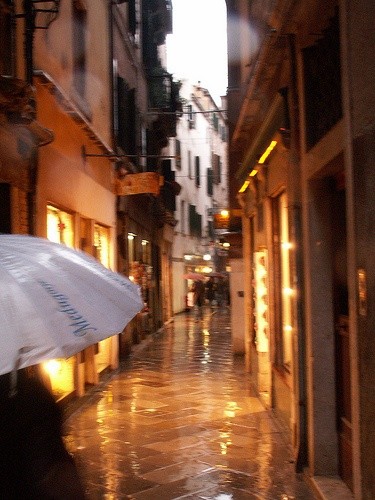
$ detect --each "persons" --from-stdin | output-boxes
[205,277,215,307]
[189,278,205,322]
[1,362,71,500]
[217,279,226,306]
[225,277,230,304]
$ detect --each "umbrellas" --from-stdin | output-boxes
[1,233,143,376]
[182,272,205,281]
[204,271,227,278]
